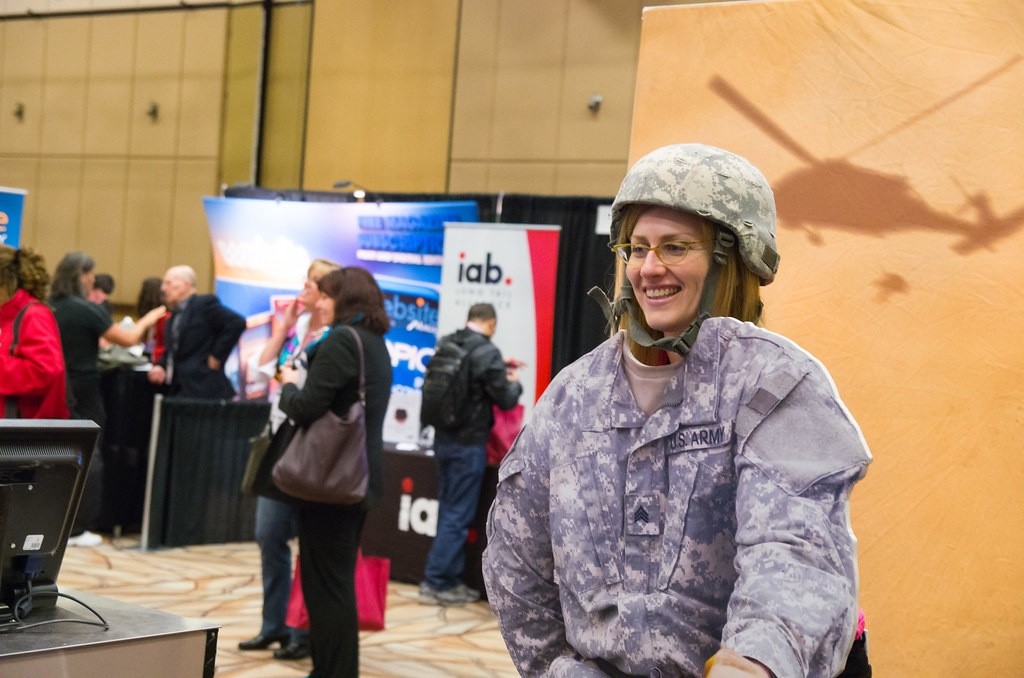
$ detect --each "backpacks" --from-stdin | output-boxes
[420,333,497,430]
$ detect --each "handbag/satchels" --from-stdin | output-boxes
[240,436,272,498]
[271,332,370,504]
[286,547,391,631]
[253,420,303,500]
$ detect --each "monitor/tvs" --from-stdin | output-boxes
[0,418,100,613]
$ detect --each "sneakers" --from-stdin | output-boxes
[419,582,480,606]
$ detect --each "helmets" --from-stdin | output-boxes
[608,143,780,286]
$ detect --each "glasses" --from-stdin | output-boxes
[612,239,711,265]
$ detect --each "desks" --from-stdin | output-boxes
[363,440,502,596]
[0,589,225,678]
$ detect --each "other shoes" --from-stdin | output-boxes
[67,530,103,548]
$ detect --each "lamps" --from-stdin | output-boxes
[334,179,377,203]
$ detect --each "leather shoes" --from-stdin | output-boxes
[239,630,292,650]
[272,635,312,660]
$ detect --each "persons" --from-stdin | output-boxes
[44,251,167,547]
[87,274,113,303]
[239,258,341,660]
[481,142,872,678]
[419,303,523,607]
[0,243,70,419]
[279,266,392,678]
[148,265,246,400]
[137,277,171,364]
